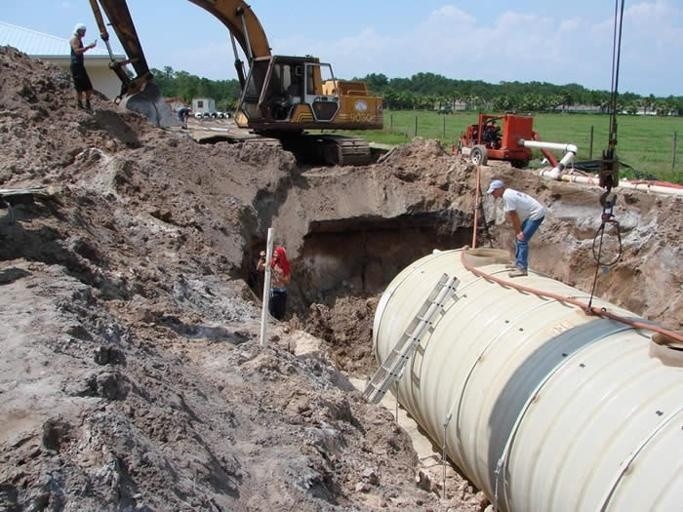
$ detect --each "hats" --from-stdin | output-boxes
[487,179,504,194]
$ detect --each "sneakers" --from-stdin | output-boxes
[509,270,528,277]
[506,263,517,268]
[78,103,90,109]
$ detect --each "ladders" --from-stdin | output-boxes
[361,273,459,404]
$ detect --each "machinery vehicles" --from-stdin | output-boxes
[460,112,534,167]
[87,0,385,165]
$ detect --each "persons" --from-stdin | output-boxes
[67,23,98,110]
[175,106,192,129]
[483,118,499,149]
[491,121,502,147]
[254,246,291,320]
[485,179,545,278]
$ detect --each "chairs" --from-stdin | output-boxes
[282,76,302,103]
